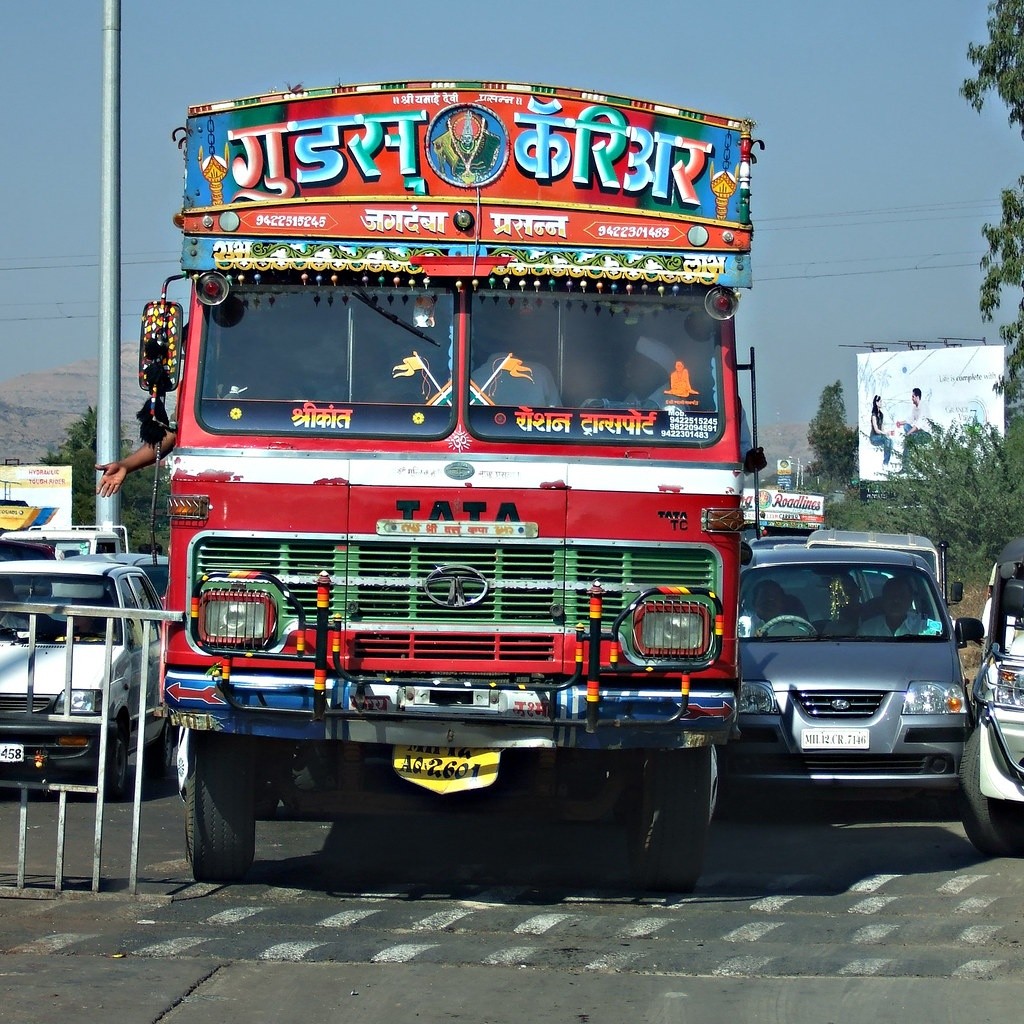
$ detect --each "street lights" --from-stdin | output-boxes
[785,456,802,489]
[790,462,804,488]
[807,460,819,487]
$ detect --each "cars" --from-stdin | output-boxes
[64,550,169,630]
[957,537,1024,862]
[0,538,56,638]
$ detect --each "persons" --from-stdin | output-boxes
[870,389,931,476]
[739,578,808,638]
[94,323,305,495]
[855,576,928,636]
[472,322,564,409]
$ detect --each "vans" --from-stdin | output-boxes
[0,559,170,804]
[741,528,965,628]
[720,547,986,813]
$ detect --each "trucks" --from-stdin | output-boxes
[0,525,130,562]
[131,76,763,895]
